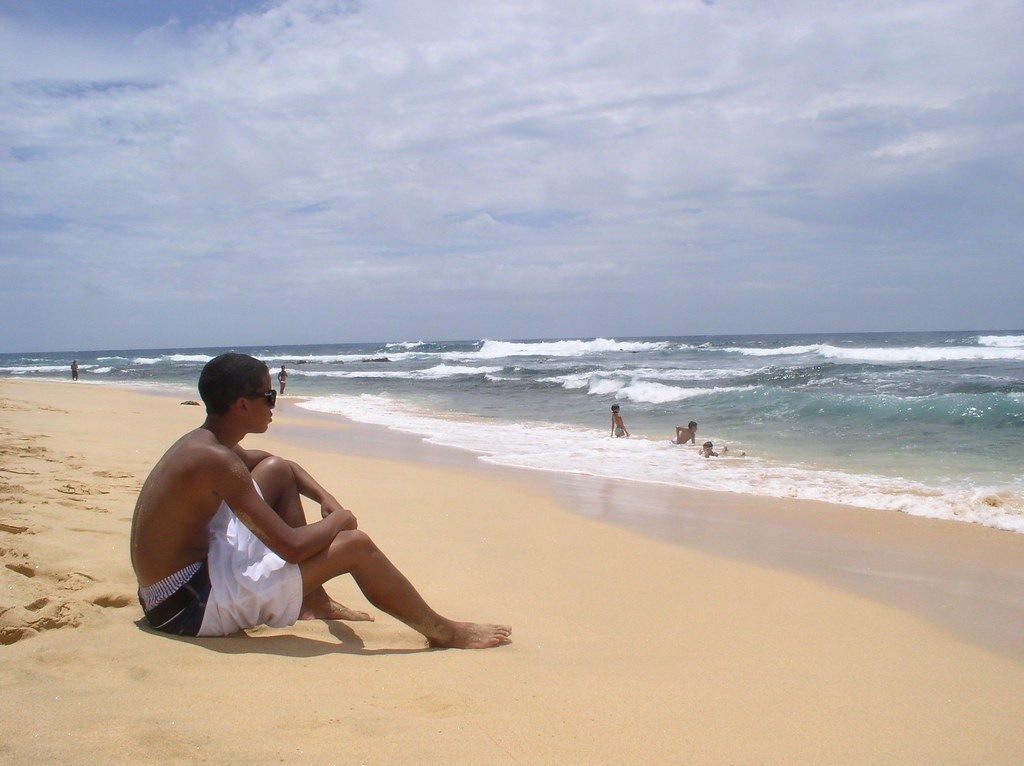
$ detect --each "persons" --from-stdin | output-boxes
[277,365,288,395]
[609,404,630,439]
[698,442,745,458]
[671,421,696,445]
[130,353,514,648]
[70,360,78,381]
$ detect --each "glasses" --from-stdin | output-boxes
[244,390,277,405]
